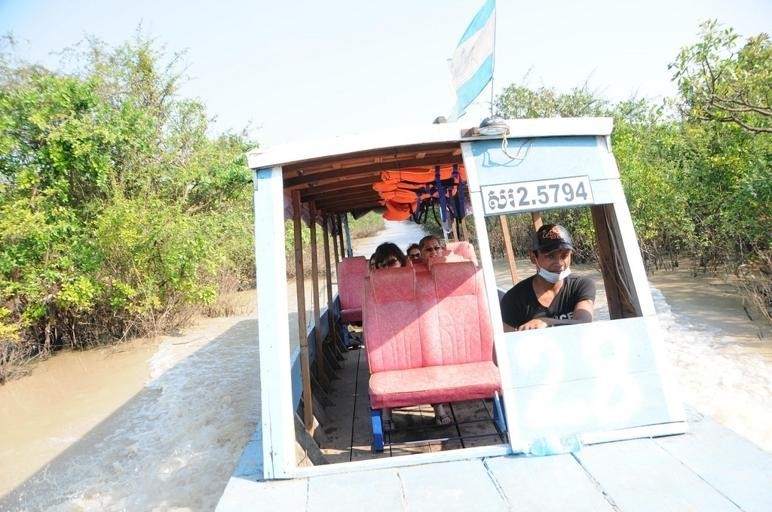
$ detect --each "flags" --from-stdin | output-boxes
[441,0,496,123]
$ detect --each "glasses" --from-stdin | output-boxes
[426,246,440,252]
[409,254,419,257]
[380,258,397,268]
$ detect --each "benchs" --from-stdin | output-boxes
[335,240,507,456]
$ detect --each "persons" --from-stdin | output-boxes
[368,235,452,433]
[491,225,596,367]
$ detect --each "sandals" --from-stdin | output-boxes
[384,416,396,432]
[435,413,452,427]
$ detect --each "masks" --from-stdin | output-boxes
[534,258,571,283]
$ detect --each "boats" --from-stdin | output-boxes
[212,117,770,512]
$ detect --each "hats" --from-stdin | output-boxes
[533,224,576,256]
